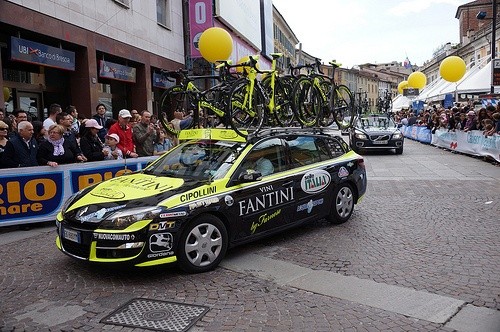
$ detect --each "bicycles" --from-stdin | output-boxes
[157,51,355,138]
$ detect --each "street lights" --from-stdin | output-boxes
[475,0,497,94]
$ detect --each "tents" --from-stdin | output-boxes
[384,54,491,113]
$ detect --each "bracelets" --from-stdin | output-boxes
[128,151,132,156]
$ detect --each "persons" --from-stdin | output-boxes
[34,124,81,167]
[3,120,34,169]
[108,109,139,158]
[239,147,274,178]
[389,100,500,167]
[0,103,225,169]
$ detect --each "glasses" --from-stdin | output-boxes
[0,128,8,131]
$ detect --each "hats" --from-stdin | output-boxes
[465,111,475,116]
[104,134,120,144]
[119,109,132,118]
[85,119,103,129]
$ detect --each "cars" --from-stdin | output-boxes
[348,113,405,155]
[54,125,367,275]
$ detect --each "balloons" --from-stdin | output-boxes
[198,26,234,63]
[407,72,427,89]
[440,55,467,82]
